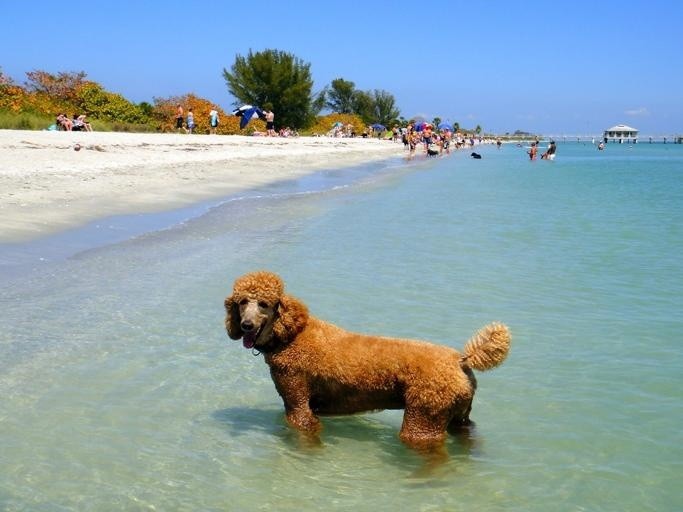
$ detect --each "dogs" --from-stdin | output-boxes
[471,152,481,160]
[224,270,511,477]
[427,149,438,157]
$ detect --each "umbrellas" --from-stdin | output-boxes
[238,107,257,130]
[233,104,265,118]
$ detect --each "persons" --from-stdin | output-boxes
[526,136,557,161]
[278,127,299,138]
[174,103,189,135]
[325,119,502,162]
[264,108,274,137]
[596,141,604,151]
[208,110,219,135]
[187,108,194,135]
[54,112,94,132]
